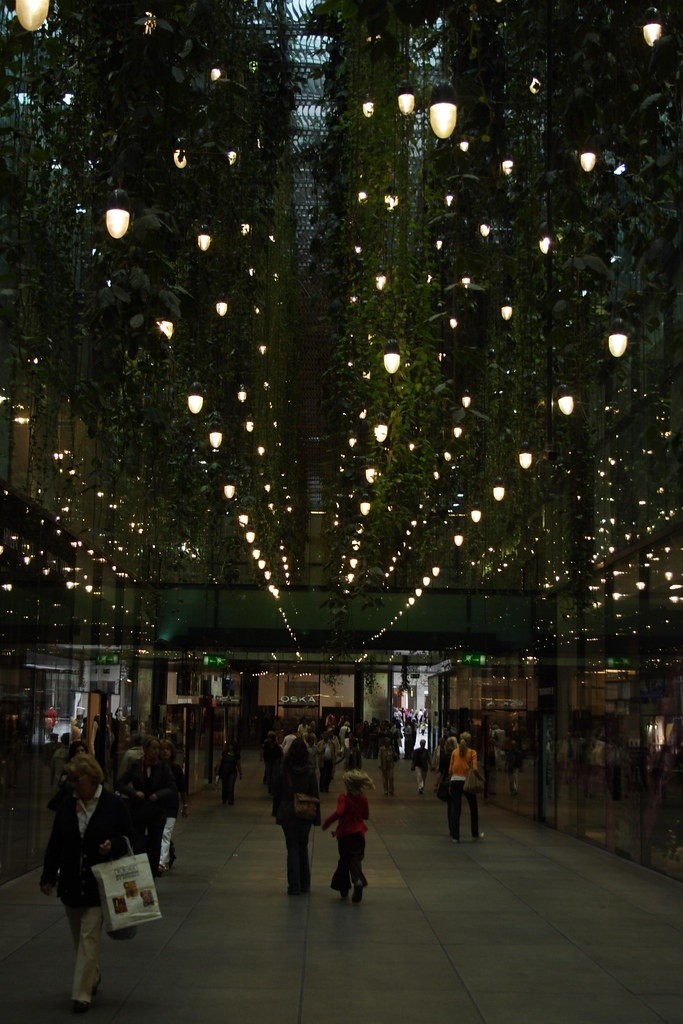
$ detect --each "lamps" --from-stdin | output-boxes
[365,465,377,485]
[384,341,401,374]
[642,7,663,46]
[217,298,227,318]
[363,95,374,117]
[209,424,223,449]
[359,495,370,517]
[107,190,131,239]
[397,82,415,115]
[609,321,628,357]
[556,386,574,415]
[500,297,513,321]
[15,0,50,31]
[373,415,388,442]
[223,475,236,499]
[518,443,532,470]
[493,477,504,502]
[579,147,597,173]
[187,381,203,414]
[197,225,211,251]
[428,84,458,138]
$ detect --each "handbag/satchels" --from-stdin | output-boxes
[91,836,163,933]
[462,769,482,793]
[294,792,320,821]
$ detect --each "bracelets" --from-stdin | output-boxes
[479,776,481,780]
[183,804,188,807]
[239,773,243,775]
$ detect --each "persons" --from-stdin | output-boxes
[39,754,135,1015]
[322,769,376,903]
[377,736,395,795]
[447,731,486,843]
[116,731,191,876]
[41,711,152,798]
[479,719,630,802]
[57,740,88,787]
[432,734,461,801]
[270,737,320,896]
[263,709,430,797]
[219,737,243,806]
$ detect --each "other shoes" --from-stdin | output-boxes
[340,890,347,896]
[157,868,162,877]
[287,886,300,894]
[74,1000,88,1012]
[300,884,309,891]
[351,881,363,902]
[473,833,484,841]
[452,839,458,843]
